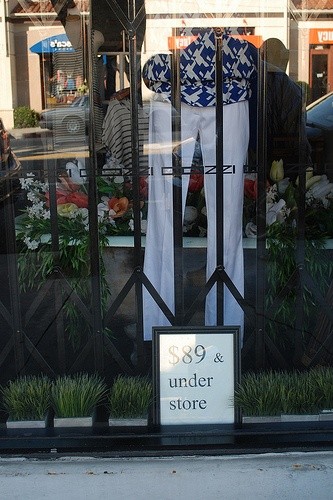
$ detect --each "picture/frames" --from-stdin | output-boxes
[151,325,243,425]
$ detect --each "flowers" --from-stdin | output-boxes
[15,159,333,351]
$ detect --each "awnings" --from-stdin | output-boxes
[29,34,77,53]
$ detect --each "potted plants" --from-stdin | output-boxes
[13,104,40,138]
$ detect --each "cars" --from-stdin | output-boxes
[170,92,333,183]
[39,95,178,137]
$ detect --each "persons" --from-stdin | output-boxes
[143,1,257,351]
[101,55,181,175]
